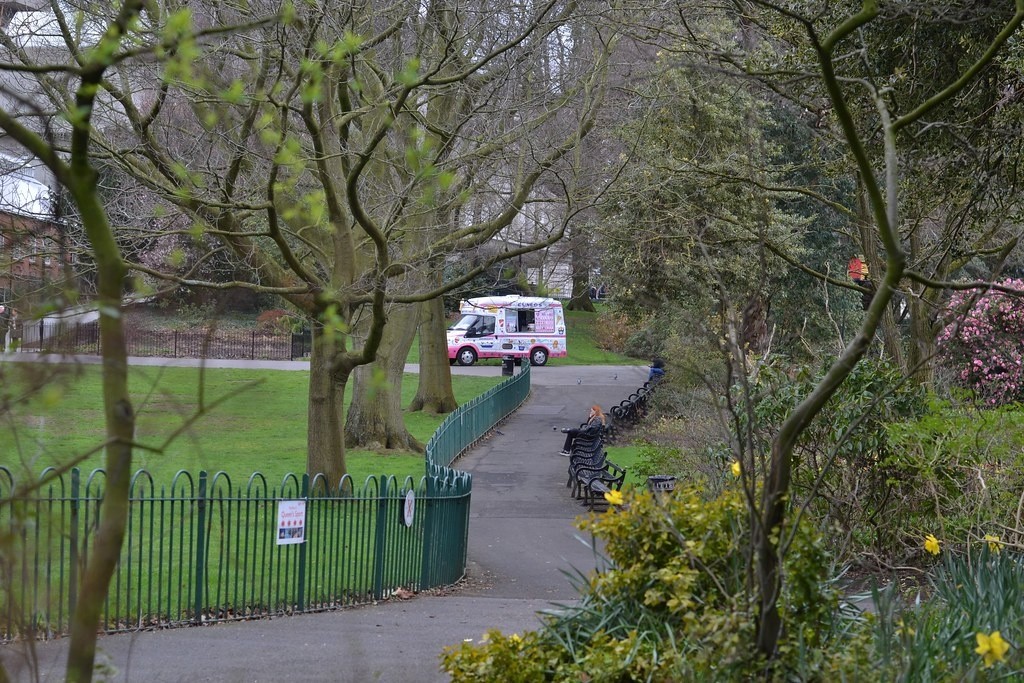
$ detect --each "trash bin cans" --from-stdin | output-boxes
[501,354,514,375]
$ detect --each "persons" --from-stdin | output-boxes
[0,306,9,352]
[559,406,605,456]
[7,309,23,353]
[280,529,302,538]
[647,358,665,387]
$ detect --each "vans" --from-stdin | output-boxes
[447,295,566,366]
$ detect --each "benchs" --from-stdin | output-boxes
[566,368,668,515]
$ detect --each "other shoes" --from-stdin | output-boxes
[560,428,571,433]
[559,450,570,456]
